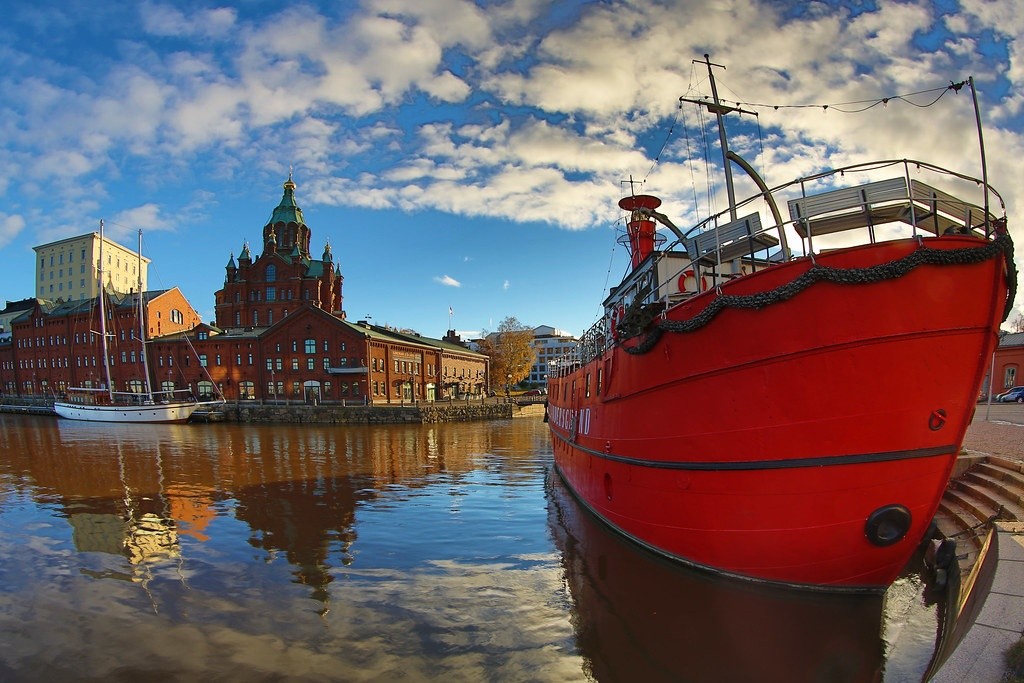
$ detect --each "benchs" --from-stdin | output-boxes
[787,176,909,257]
[900,180,997,241]
[685,212,779,294]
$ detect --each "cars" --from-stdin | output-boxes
[995,384,1024,404]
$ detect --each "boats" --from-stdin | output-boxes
[50,220,228,424]
[541,52,1019,596]
